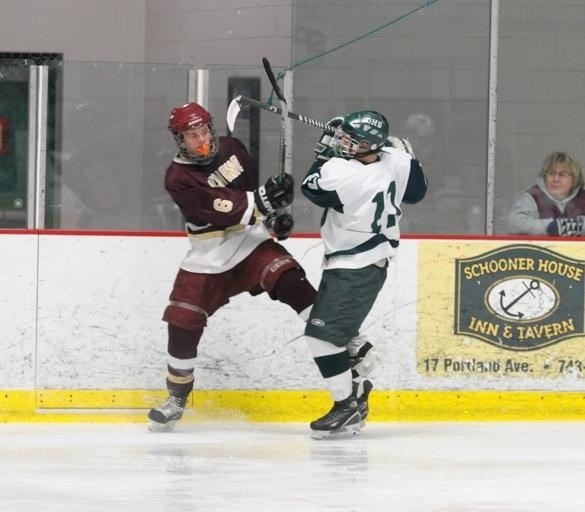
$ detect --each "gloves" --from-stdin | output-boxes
[253,172,294,241]
[312,117,344,160]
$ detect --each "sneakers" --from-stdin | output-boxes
[149,393,186,424]
[311,381,373,431]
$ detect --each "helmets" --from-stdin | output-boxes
[332,110,389,160]
[167,102,218,163]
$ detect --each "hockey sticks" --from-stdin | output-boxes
[226,94,337,134]
[262,57,288,217]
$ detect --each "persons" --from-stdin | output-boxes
[507,152,585,235]
[299,110,429,431]
[147,103,316,424]
